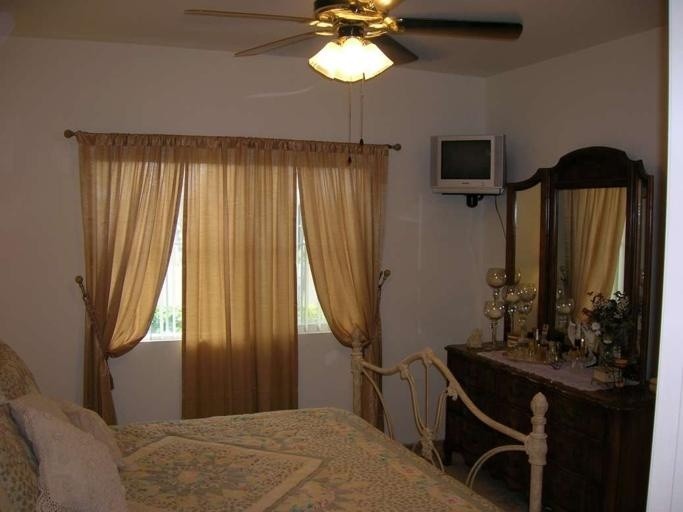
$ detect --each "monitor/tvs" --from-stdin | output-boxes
[430,133,507,194]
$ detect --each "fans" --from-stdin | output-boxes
[183,0,524,67]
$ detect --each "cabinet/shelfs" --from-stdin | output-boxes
[442,344,654,512]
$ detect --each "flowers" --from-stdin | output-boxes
[582,290,630,343]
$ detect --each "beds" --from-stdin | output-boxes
[0,319,552,512]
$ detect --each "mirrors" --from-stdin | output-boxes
[503,165,541,344]
[550,146,639,346]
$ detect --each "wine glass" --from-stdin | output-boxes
[556,295,577,318]
[485,270,506,300]
[483,302,505,349]
[502,269,539,351]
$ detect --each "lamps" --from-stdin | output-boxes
[310,37,393,83]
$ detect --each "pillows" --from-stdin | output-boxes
[63,404,126,472]
[4,392,69,437]
[21,406,128,511]
